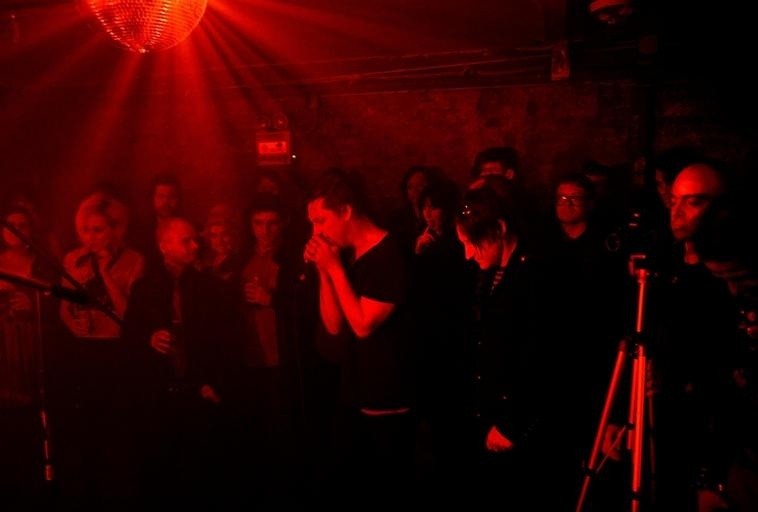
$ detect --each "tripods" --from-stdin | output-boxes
[575,281,656,512]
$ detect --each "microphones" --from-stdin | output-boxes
[47,284,96,306]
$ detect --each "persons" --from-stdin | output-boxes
[0,146,757,512]
